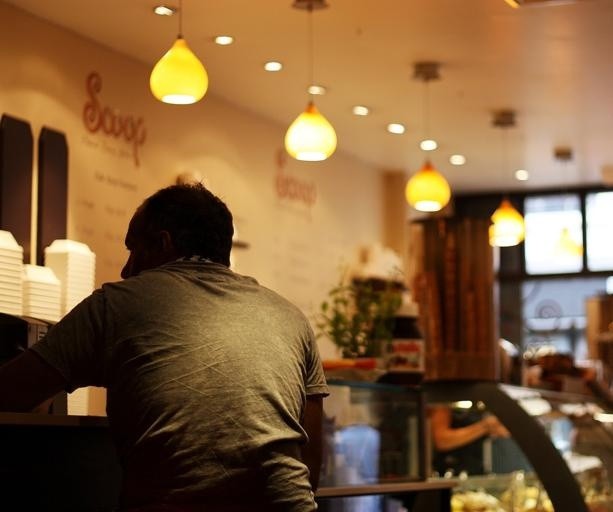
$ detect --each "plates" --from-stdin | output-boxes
[22,264,63,324]
[0,229,25,318]
[42,237,96,416]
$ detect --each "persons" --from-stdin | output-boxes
[0,183,329,511]
[424,400,512,476]
[368,353,430,482]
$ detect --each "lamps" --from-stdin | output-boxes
[283,1,337,162]
[487,110,527,247]
[149,2,209,105]
[404,62,451,212]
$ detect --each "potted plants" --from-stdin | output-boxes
[313,273,407,380]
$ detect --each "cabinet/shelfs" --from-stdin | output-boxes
[308,377,613,512]
[584,293,612,398]
